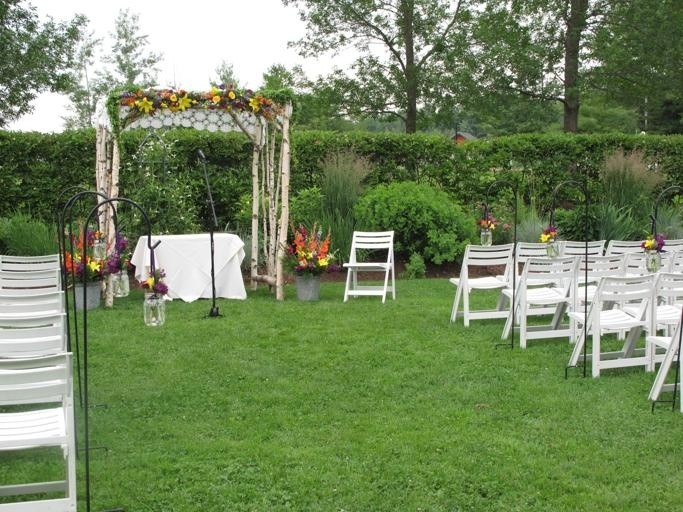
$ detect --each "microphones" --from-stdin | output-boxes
[198,149,205,159]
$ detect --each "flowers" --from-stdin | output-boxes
[280,221,343,275]
[63,226,129,283]
[131,85,280,121]
[477,206,498,230]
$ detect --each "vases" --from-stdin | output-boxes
[73,282,100,310]
[140,267,168,295]
[294,272,320,301]
[143,293,165,327]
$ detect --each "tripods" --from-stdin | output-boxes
[188,159,227,321]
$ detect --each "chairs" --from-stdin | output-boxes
[0,253,78,512]
[342,230,396,303]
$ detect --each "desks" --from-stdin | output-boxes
[131,234,249,301]
